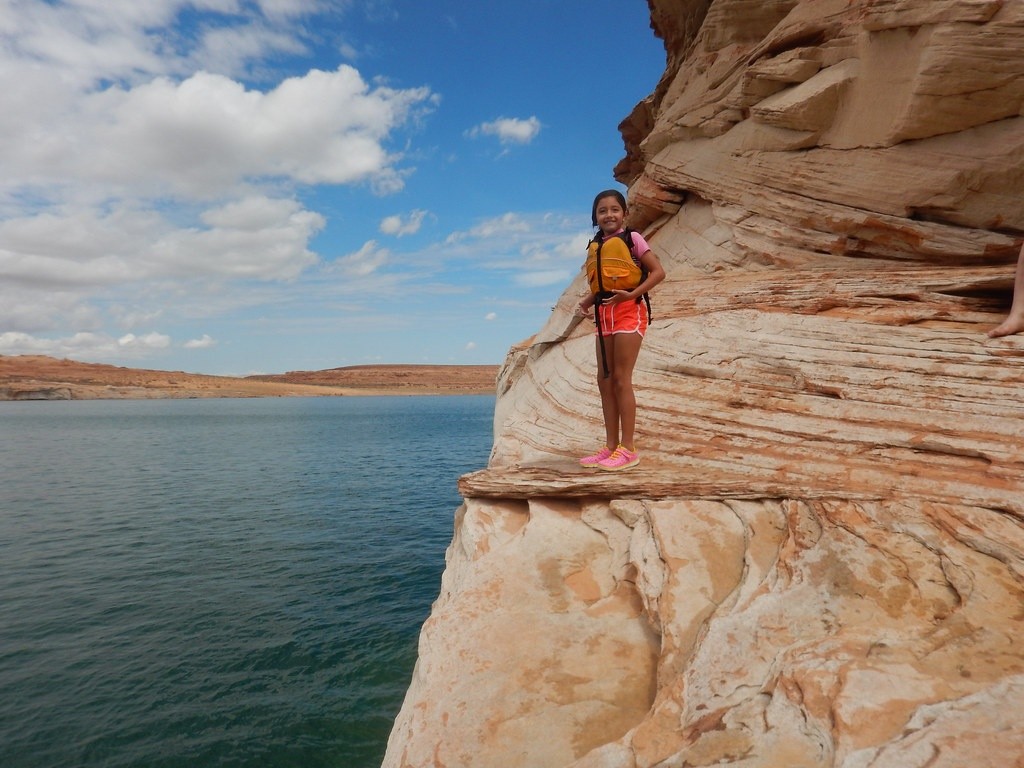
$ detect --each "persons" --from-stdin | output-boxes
[989,242,1024,336]
[579,189,667,471]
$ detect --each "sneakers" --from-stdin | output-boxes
[598,444,639,471]
[579,446,613,468]
